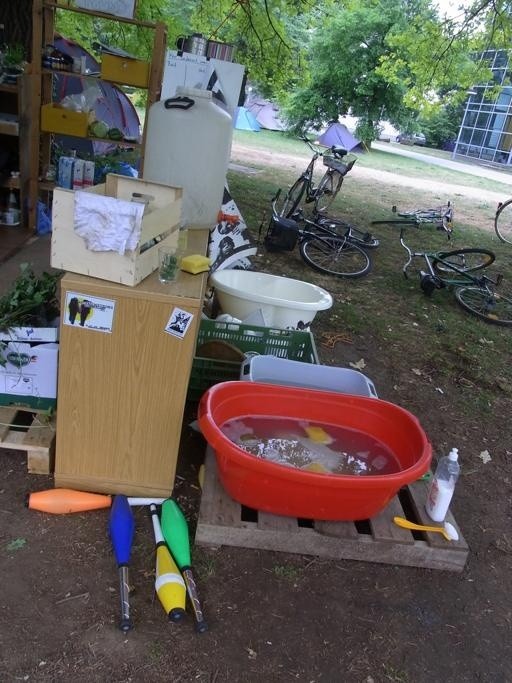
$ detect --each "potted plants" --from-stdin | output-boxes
[3,41,28,84]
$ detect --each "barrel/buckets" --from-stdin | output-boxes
[141,85,235,229]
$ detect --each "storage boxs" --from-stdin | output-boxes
[40,103,95,139]
[48,173,183,288]
[98,51,152,88]
[0,326,59,413]
[73,0,136,19]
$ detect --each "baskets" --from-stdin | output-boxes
[265,215,299,252]
[323,145,358,176]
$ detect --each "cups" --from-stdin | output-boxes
[158,245,184,287]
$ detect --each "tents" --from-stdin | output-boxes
[235,95,286,135]
[54,30,141,160]
[312,123,365,153]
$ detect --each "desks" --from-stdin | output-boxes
[54,227,211,499]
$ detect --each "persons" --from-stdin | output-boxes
[80,299,92,326]
[216,235,234,262]
[169,312,189,332]
[68,297,80,325]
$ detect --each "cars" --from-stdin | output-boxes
[396,130,426,146]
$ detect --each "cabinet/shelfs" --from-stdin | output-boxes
[0,0,56,232]
[28,0,170,238]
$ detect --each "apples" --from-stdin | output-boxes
[90,120,110,139]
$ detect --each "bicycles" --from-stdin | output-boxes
[282,129,360,219]
[395,227,512,326]
[496,196,512,244]
[371,199,456,239]
[258,188,381,277]
